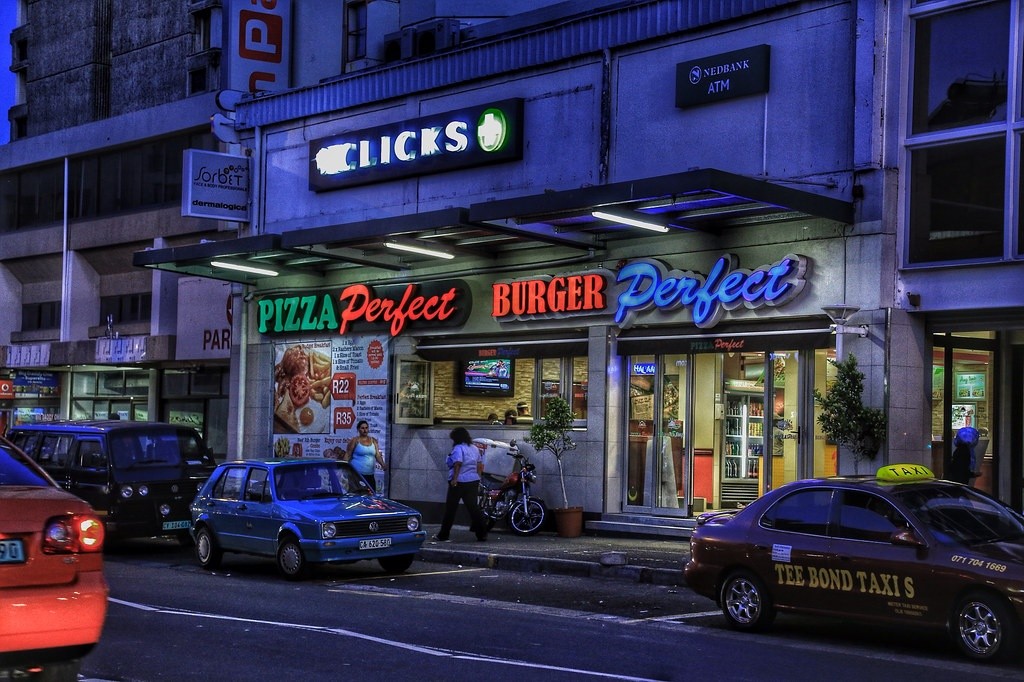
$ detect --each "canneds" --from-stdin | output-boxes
[725,400,764,454]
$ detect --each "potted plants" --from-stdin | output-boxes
[521,395,585,539]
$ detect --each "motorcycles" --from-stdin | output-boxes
[477,437,549,537]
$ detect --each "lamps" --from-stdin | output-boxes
[822,306,869,339]
[211,257,279,277]
[383,237,455,259]
[592,208,670,234]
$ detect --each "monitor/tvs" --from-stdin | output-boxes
[458,359,515,398]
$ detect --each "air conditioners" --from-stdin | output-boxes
[417,19,460,54]
[380,28,417,63]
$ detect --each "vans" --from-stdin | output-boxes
[6,418,219,553]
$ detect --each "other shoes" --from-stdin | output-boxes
[479,537,487,542]
[432,534,440,540]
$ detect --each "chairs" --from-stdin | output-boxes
[81,441,100,468]
[147,443,176,463]
[301,473,327,497]
[899,497,924,532]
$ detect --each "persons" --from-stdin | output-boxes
[473,360,504,376]
[345,420,388,495]
[487,401,529,425]
[431,427,488,541]
[318,446,348,495]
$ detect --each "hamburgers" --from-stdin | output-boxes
[281,344,309,379]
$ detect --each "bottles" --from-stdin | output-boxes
[727,401,742,415]
[749,444,761,454]
[725,459,738,477]
[747,459,758,478]
[725,442,739,455]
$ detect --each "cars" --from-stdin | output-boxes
[683,464,1024,667]
[190,457,427,581]
[0,435,109,653]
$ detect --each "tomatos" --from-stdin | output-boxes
[289,375,310,406]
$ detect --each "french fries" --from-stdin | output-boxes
[305,351,332,409]
